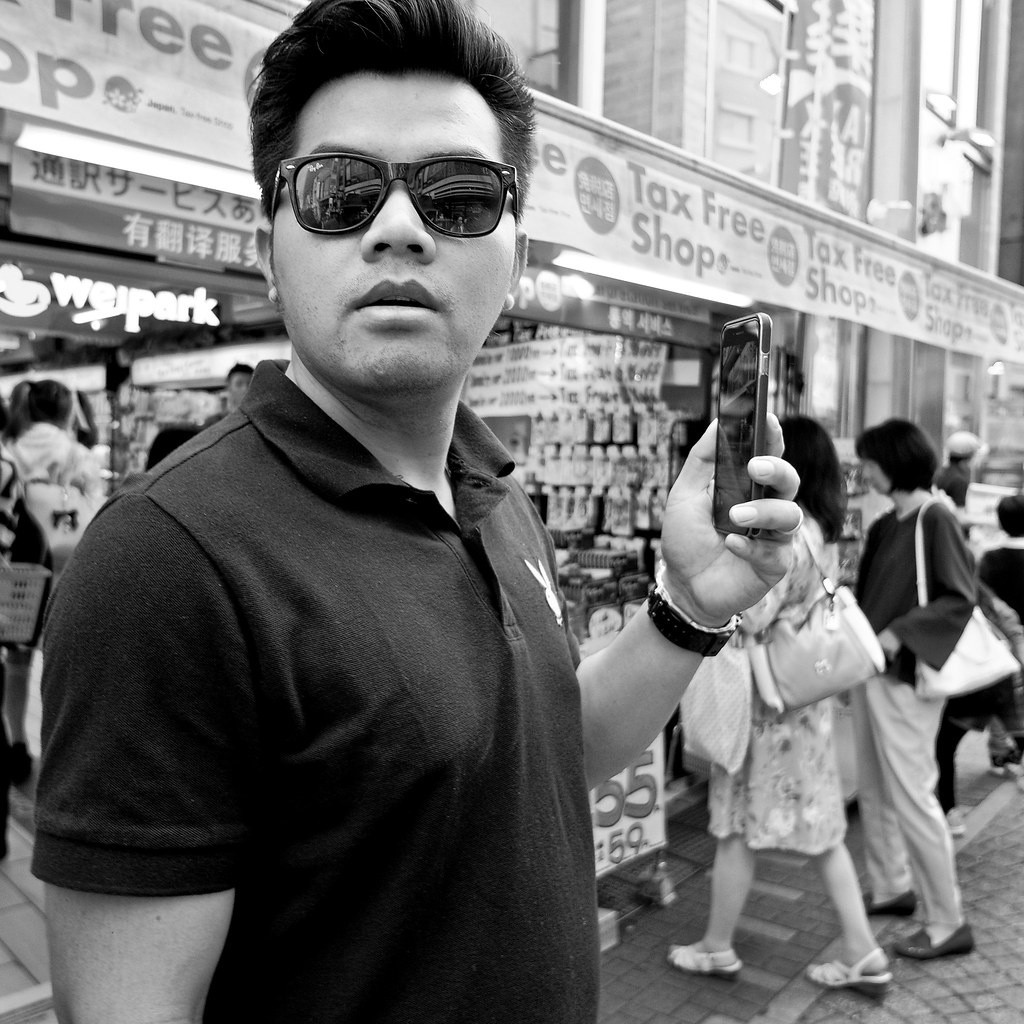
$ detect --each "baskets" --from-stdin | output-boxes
[0,553,52,643]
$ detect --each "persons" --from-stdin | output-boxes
[670,416,894,994]
[855,420,976,958]
[934,428,1024,837]
[33,0,804,1023]
[1,365,256,861]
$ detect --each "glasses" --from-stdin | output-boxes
[271,153,518,238]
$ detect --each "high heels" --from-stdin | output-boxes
[806,947,893,995]
[666,945,742,980]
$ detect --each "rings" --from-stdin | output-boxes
[777,505,804,537]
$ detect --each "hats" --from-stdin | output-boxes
[947,431,983,456]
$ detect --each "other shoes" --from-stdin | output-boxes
[894,923,974,959]
[946,808,967,834]
[863,890,916,917]
[9,742,31,785]
[991,746,1024,776]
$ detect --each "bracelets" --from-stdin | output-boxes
[648,565,743,658]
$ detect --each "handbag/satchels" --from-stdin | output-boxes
[914,498,1022,698]
[680,629,754,779]
[24,442,109,564]
[747,525,885,714]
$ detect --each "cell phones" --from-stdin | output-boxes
[712,313,772,538]
